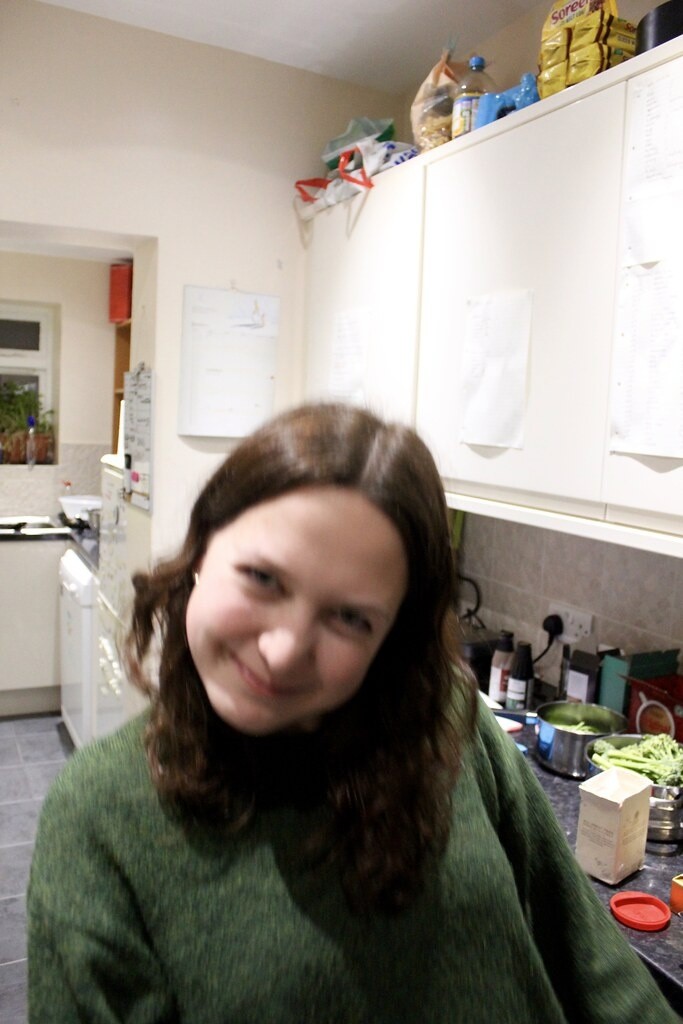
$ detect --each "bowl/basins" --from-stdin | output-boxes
[59,496,102,524]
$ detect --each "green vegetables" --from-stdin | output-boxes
[588,732,683,787]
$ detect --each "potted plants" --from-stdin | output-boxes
[0,382,53,464]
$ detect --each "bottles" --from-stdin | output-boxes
[60,481,74,498]
[452,56,499,139]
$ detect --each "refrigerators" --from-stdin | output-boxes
[93,454,150,737]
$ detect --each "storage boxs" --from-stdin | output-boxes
[574,767,653,885]
[565,647,620,704]
[618,673,683,743]
[109,264,133,323]
[599,649,680,719]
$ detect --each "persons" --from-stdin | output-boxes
[18,400,681,1024]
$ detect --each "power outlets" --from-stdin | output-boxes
[548,603,593,648]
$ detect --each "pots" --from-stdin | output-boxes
[533,699,629,778]
[584,734,683,856]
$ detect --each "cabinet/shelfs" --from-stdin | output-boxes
[112,318,131,455]
[416,33,682,560]
[298,155,417,430]
